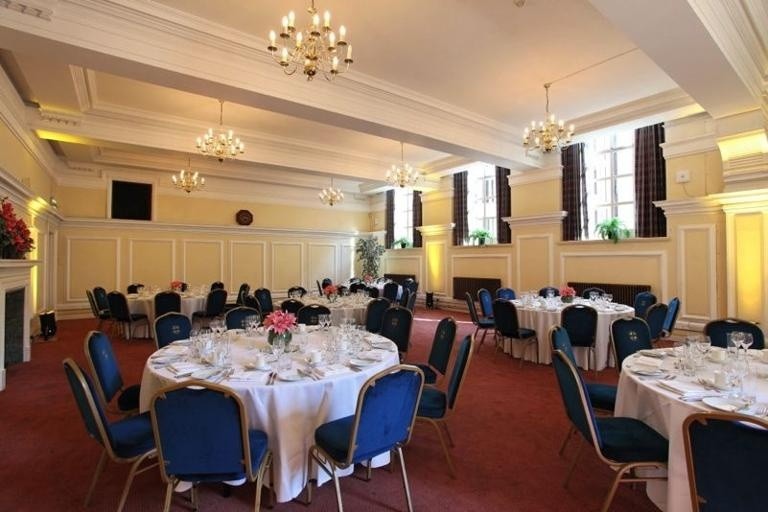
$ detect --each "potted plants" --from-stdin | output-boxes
[469,231,494,245]
[392,237,409,248]
[594,216,631,243]
[356,235,385,284]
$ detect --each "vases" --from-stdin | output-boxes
[9,249,25,259]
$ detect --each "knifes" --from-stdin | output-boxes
[265,372,278,386]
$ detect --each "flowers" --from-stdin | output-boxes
[0,197,35,252]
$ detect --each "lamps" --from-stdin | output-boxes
[172,158,205,193]
[319,177,345,207]
[195,100,245,164]
[267,1,353,82]
[384,143,420,187]
[522,83,576,152]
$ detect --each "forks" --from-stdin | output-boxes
[212,368,234,384]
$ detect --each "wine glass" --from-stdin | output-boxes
[519,288,614,309]
[137,282,207,296]
[310,288,370,303]
[188,315,367,370]
[673,331,753,376]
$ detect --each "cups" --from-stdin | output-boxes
[714,369,728,386]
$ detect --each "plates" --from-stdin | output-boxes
[191,371,211,380]
[350,359,376,367]
[152,356,175,364]
[278,371,304,381]
[630,365,669,376]
[702,397,743,413]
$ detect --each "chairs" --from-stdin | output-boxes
[682,412,768,512]
[466,287,680,373]
[550,325,618,455]
[551,350,669,512]
[703,318,765,350]
